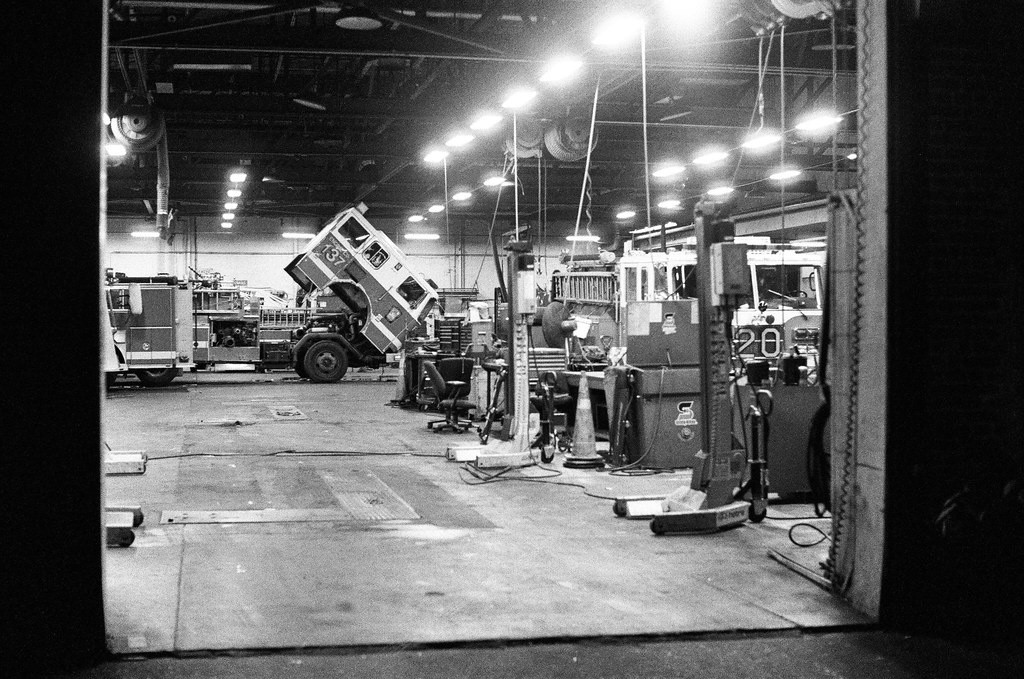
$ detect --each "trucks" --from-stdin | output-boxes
[555,237,828,404]
[99,205,438,388]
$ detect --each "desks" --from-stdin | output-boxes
[564,366,619,442]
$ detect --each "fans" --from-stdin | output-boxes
[543,302,578,368]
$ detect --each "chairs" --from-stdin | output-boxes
[428,357,474,399]
[423,361,482,435]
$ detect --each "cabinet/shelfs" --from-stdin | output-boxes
[437,316,465,350]
[460,320,493,352]
